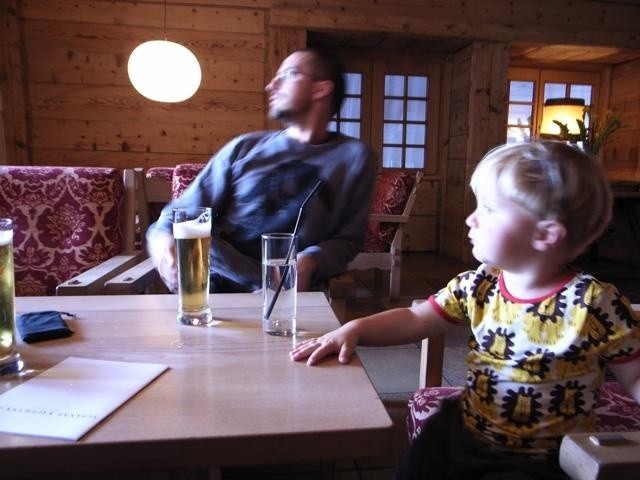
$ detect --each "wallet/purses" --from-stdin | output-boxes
[16,311,75,344]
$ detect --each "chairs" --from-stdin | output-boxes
[0,164,208,297]
[411,290,640,399]
[320,168,426,324]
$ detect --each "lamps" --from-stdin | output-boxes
[541,98,588,141]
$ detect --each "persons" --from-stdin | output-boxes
[289,140,640,480]
[146,49,374,295]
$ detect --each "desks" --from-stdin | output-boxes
[1,291,393,475]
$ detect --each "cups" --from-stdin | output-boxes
[172,205,214,327]
[0,216,25,377]
[261,232,299,338]
